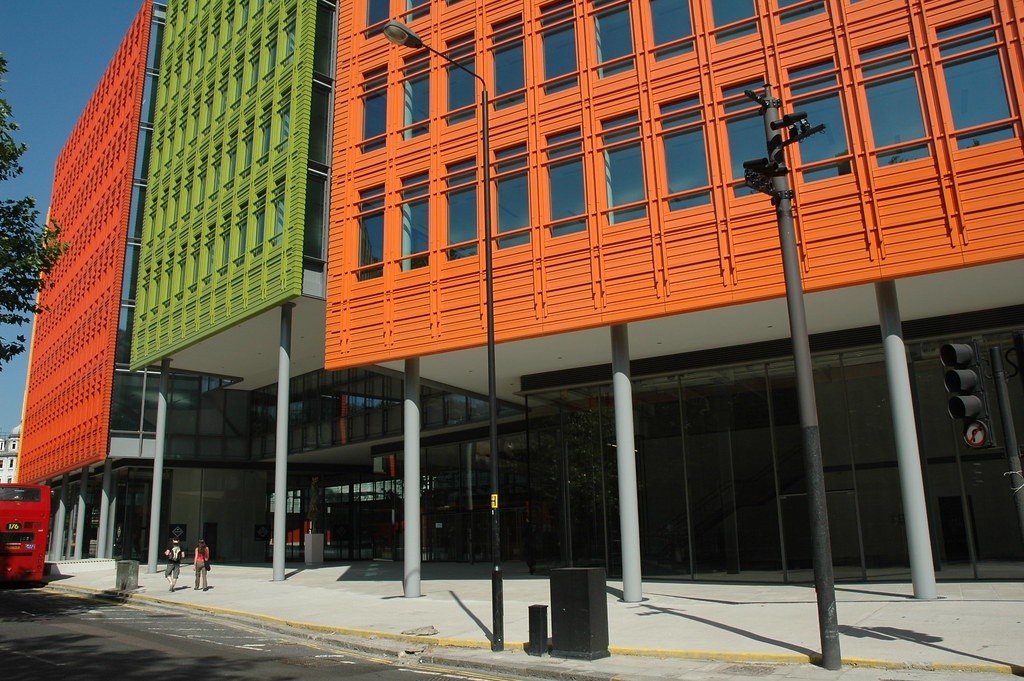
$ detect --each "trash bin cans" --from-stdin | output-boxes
[550,567,610,661]
[116,560,139,590]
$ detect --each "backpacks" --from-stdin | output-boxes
[169,542,181,561]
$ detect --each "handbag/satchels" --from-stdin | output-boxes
[204,561,211,571]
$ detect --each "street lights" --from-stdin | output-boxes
[377,18,510,654]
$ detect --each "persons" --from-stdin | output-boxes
[193,539,210,590]
[165,537,185,592]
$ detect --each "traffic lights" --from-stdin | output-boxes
[940,336,987,421]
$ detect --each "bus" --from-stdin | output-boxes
[0,481,51,583]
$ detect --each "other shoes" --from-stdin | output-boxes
[194,587,198,590]
[203,588,208,591]
[169,584,175,592]
